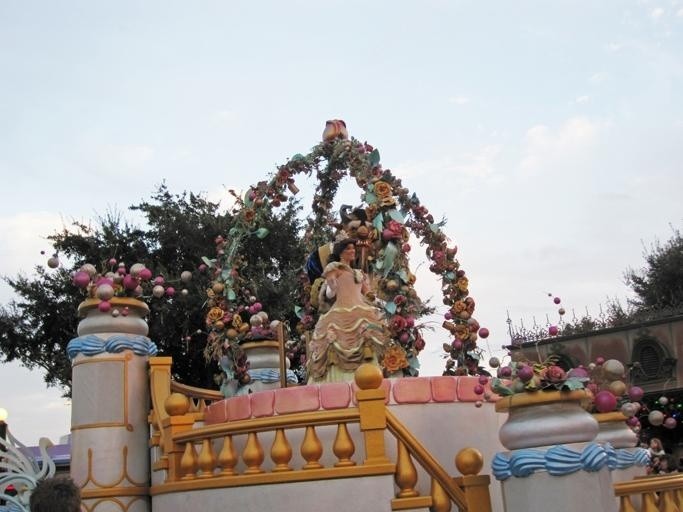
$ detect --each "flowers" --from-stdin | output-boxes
[80,271,140,298]
[491,334,590,396]
[199,117,487,385]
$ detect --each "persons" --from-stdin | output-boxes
[638,429,682,475]
[307,238,409,385]
[29,474,85,511]
